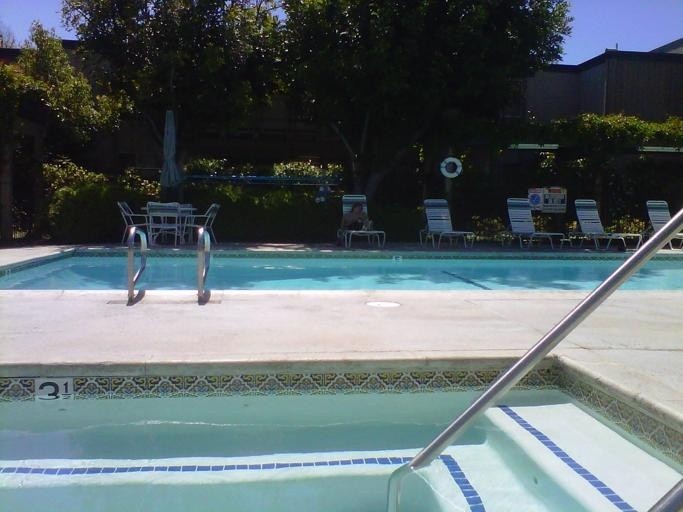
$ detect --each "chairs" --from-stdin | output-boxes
[418,199,476,250]
[501,198,683,251]
[338,194,386,249]
[117,200,220,247]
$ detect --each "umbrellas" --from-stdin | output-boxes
[160,108,183,202]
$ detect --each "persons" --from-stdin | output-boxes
[340,202,371,231]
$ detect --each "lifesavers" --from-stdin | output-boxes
[440,157,462,179]
